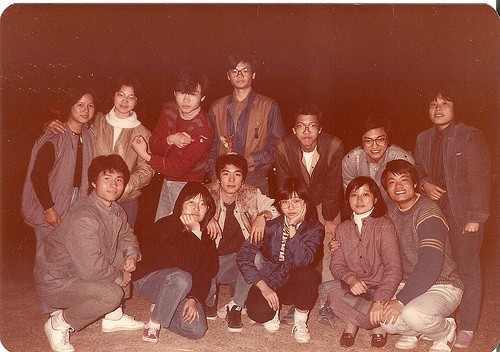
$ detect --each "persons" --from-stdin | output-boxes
[236,176,325,343]
[33,155,145,352]
[43,79,155,308]
[328,159,464,352]
[208,51,286,196]
[130,183,219,343]
[22,84,97,314]
[414,84,491,349]
[274,104,344,325]
[203,155,281,332]
[342,114,415,199]
[327,176,403,348]
[133,69,215,236]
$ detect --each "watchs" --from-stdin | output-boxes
[257,213,267,221]
[374,301,384,305]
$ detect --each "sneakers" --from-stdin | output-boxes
[264,308,280,331]
[395,333,424,349]
[204,300,217,319]
[226,305,244,333]
[43,309,74,352]
[291,319,310,344]
[429,333,456,352]
[101,316,145,332]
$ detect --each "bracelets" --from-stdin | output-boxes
[188,297,198,303]
[288,224,297,228]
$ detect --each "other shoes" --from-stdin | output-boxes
[455,332,474,348]
[371,334,387,349]
[141,322,159,344]
[217,301,242,318]
[318,307,335,325]
[281,305,296,324]
[340,326,358,346]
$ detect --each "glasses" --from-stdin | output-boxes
[362,137,388,147]
[229,68,253,78]
[296,125,320,132]
